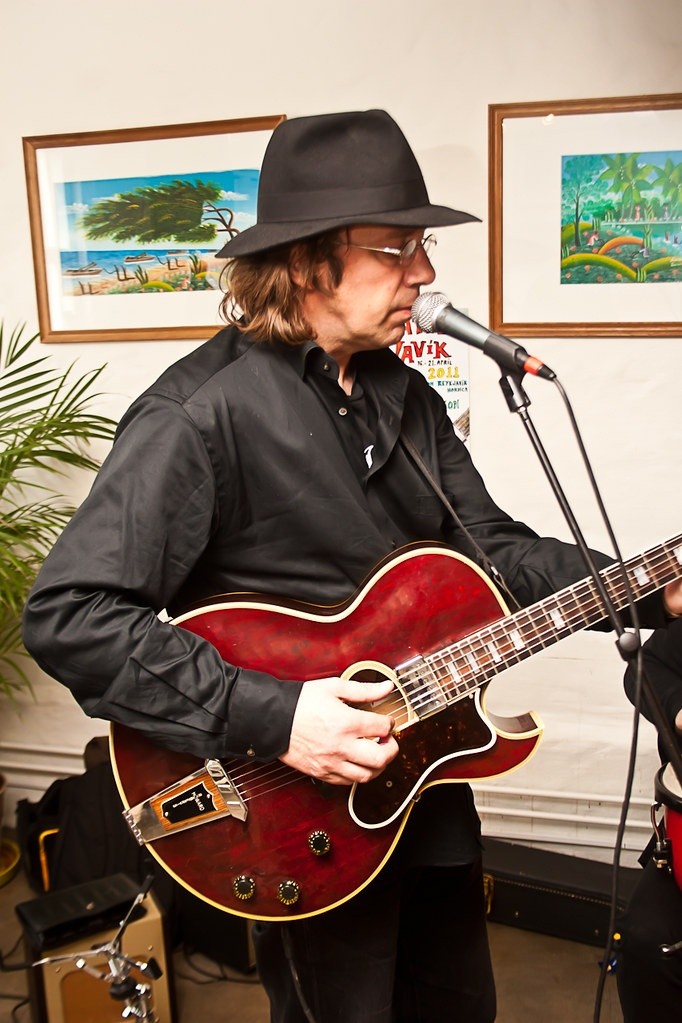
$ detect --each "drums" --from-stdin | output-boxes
[654,758,681,900]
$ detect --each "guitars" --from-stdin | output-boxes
[104,534,681,926]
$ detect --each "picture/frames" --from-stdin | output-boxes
[22,114,287,344]
[488,92,682,339]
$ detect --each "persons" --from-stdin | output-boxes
[21,110,682,1023]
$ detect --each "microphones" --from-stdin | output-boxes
[411,292,557,381]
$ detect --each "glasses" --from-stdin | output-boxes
[326,233,438,267]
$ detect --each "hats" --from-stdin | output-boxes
[213,108,484,259]
